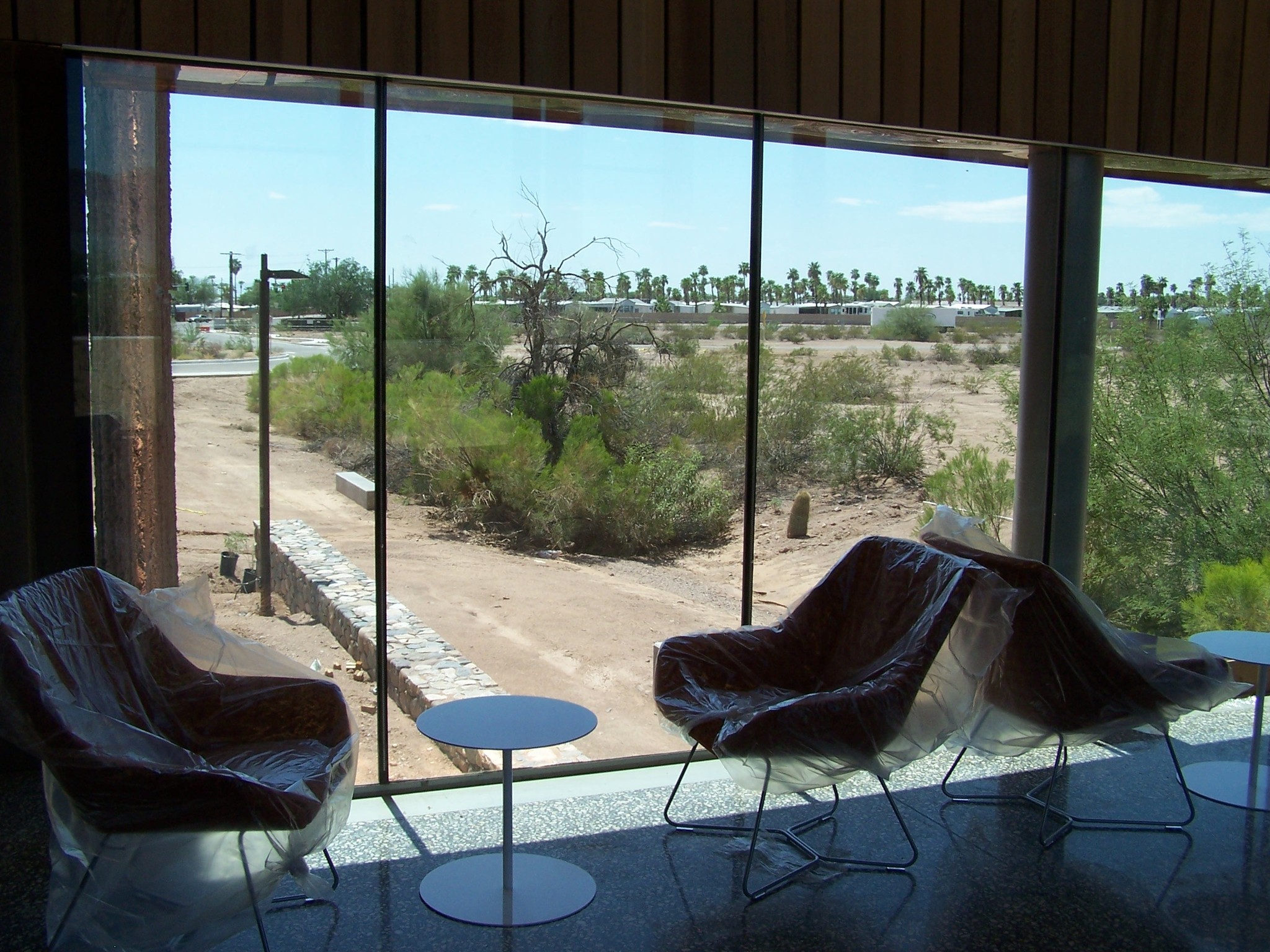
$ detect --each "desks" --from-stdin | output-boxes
[1176,631,1270,810]
[416,695,597,927]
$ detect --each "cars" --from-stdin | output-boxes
[186,314,213,323]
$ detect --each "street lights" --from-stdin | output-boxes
[220,251,242,319]
[261,252,312,616]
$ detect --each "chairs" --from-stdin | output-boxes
[653,537,978,901]
[0,566,352,952]
[922,532,1228,848]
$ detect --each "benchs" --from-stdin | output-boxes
[282,319,345,330]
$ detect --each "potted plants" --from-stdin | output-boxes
[220,529,248,576]
[240,541,256,594]
